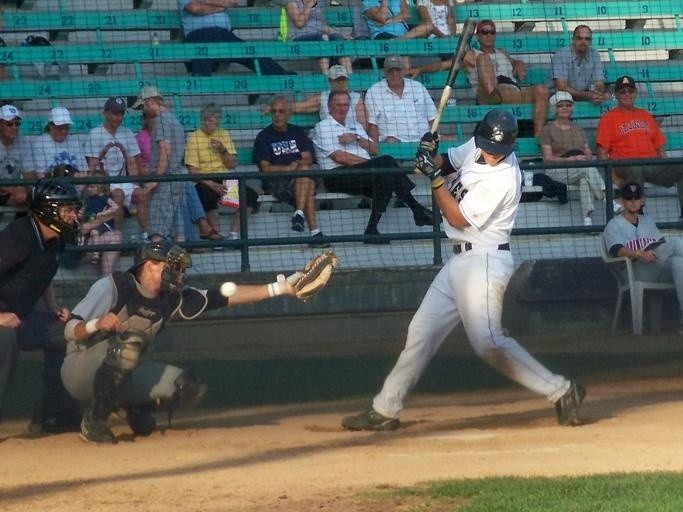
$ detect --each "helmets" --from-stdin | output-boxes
[32,182,83,236]
[475,109,519,154]
[132,239,191,293]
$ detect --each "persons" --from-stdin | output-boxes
[343,108,586,432]
[603,182,683,315]
[1,180,85,438]
[60,240,306,444]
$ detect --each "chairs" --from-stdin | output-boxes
[596,231,683,338]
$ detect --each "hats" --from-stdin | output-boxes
[131,86,162,110]
[574,25,592,33]
[549,91,575,104]
[384,55,406,68]
[326,64,349,79]
[48,106,73,126]
[103,96,128,112]
[0,104,22,122]
[616,76,638,90]
[476,19,495,32]
[621,182,644,198]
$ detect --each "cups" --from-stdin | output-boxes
[524,170,534,187]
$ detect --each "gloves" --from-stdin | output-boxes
[414,131,444,180]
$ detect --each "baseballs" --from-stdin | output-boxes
[220,282,237,298]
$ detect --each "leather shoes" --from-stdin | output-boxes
[413,210,443,226]
[362,230,390,245]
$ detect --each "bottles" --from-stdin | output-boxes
[151,32,160,45]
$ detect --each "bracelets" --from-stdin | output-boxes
[268,282,281,298]
[86,319,99,334]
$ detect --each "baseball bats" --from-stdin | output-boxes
[415,18,476,174]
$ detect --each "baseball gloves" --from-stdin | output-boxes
[293,252,338,301]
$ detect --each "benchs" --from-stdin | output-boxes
[0,1,683,213]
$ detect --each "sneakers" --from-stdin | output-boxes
[292,214,306,233]
[310,231,332,248]
[487,88,505,104]
[125,407,159,435]
[78,418,116,446]
[186,228,244,254]
[556,379,588,425]
[340,406,400,432]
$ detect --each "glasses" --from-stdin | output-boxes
[270,109,286,114]
[574,36,592,41]
[0,121,21,127]
[555,104,573,107]
[479,29,496,35]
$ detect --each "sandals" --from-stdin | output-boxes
[583,216,601,236]
[603,197,625,214]
